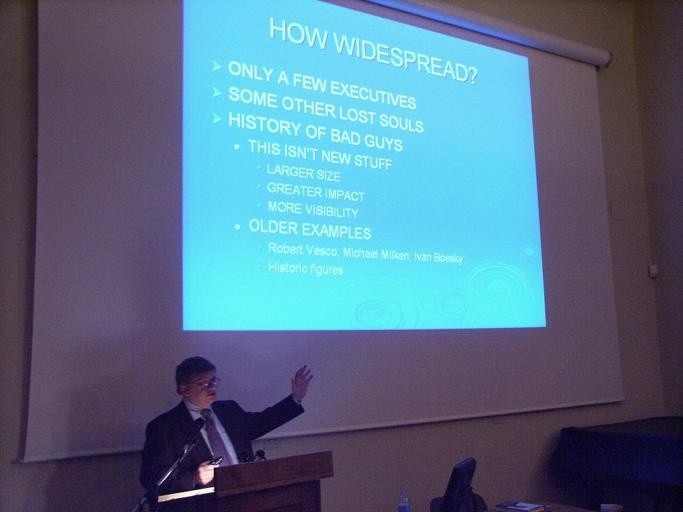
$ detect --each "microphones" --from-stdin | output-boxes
[172,417,205,458]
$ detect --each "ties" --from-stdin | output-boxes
[200,408,233,467]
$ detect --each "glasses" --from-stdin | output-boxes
[193,377,217,389]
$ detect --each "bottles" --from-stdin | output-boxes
[398,488,410,512]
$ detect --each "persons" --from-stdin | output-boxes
[253,449,265,462]
[139,357,313,491]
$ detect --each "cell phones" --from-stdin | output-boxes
[208,456,223,465]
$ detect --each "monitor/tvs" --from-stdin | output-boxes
[440,457,476,512]
